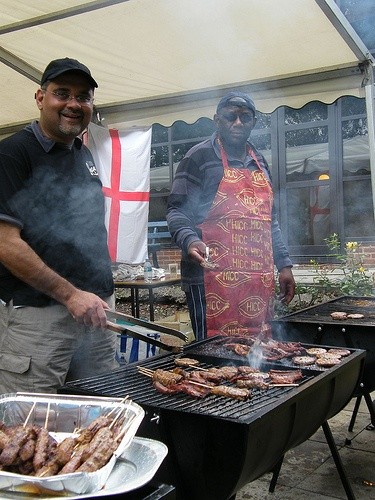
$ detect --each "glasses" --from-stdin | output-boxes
[43,88,95,105]
[224,112,252,123]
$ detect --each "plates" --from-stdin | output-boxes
[0,392,145,495]
[0,436,168,500]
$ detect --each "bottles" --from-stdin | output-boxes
[144,259,152,284]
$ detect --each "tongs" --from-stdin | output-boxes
[104,309,189,353]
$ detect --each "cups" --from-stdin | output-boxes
[169,264,178,279]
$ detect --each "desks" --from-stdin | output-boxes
[115,272,182,323]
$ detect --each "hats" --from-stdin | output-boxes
[40,57,99,88]
[216,93,256,119]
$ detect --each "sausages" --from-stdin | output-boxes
[0,416,119,475]
[237,379,269,390]
[211,386,250,400]
[152,369,182,386]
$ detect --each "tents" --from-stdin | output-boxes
[152,133,372,190]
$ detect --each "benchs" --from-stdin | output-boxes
[147,221,182,269]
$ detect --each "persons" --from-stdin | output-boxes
[166,92,296,342]
[0,56,119,396]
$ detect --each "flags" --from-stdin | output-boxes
[87,122,152,265]
